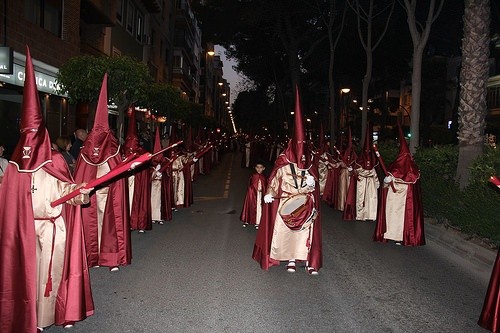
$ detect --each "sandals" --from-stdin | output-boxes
[305,265,319,275]
[286,261,296,272]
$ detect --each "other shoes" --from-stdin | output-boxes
[172,208,178,212]
[255,224,258,230]
[395,240,402,245]
[152,220,155,224]
[110,266,119,273]
[242,223,249,228]
[138,230,145,233]
[93,265,100,268]
[37,327,43,333]
[158,221,164,225]
[63,321,74,329]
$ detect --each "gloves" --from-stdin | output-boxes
[388,172,395,181]
[193,157,199,162]
[324,161,329,165]
[264,194,274,203]
[347,166,353,171]
[384,176,393,184]
[306,175,315,187]
[156,171,162,177]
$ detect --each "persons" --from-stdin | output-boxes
[0,124,426,234]
[0,126,95,333]
[253,138,329,276]
[342,147,380,223]
[73,129,131,272]
[240,162,270,230]
[372,144,426,247]
